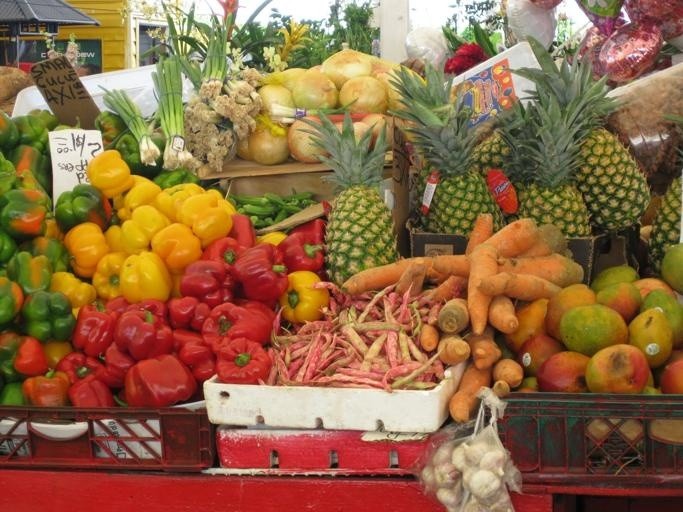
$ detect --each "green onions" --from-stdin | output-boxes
[99,0,264,173]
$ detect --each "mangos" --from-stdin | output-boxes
[514,244,683,393]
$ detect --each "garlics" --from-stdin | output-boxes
[422,442,511,512]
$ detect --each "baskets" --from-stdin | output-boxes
[490,387,683,489]
[1,404,218,474]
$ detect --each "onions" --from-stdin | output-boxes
[236,49,420,164]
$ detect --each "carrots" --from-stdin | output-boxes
[340,213,584,426]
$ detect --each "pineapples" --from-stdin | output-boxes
[324,104,683,289]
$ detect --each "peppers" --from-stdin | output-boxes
[1,109,329,421]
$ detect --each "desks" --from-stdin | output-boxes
[0,459,682,512]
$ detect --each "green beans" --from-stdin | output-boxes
[256,281,445,393]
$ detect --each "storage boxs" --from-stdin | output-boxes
[197,153,418,237]
[399,215,611,290]
